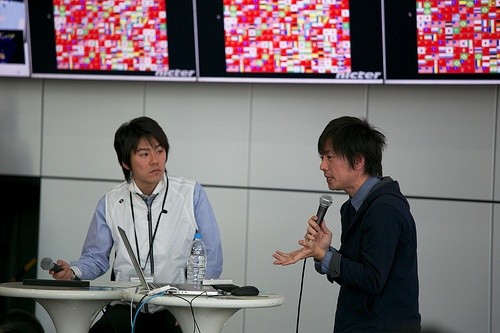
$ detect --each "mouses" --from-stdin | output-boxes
[231,286,259,296]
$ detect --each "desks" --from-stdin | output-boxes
[0,282,284,333]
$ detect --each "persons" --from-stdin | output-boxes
[273,116,421,333]
[49,116,223,333]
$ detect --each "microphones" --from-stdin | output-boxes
[310,195,334,237]
[40,257,81,281]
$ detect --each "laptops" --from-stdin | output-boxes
[118,226,219,295]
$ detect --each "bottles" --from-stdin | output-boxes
[187,233,207,291]
